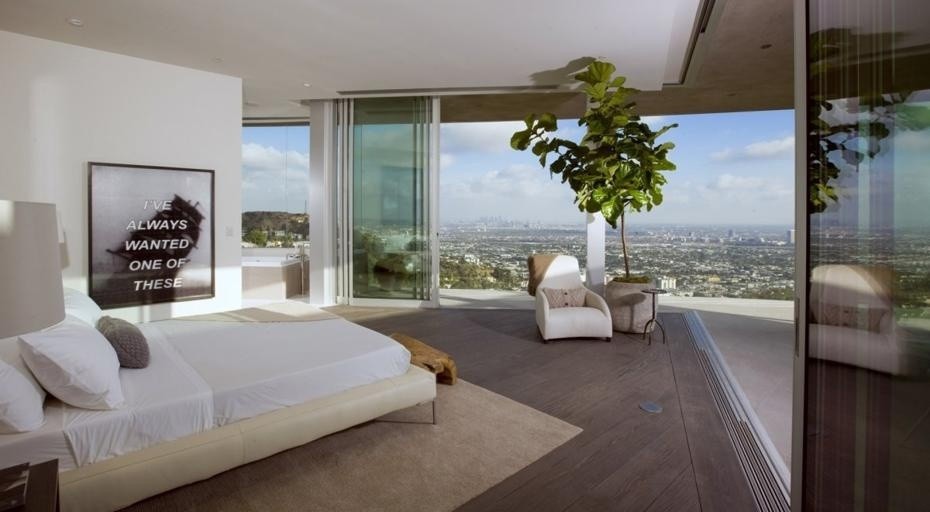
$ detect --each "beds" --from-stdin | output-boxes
[2,303,452,510]
[807,260,929,378]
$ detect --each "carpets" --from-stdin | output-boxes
[115,377,591,510]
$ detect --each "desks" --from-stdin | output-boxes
[638,288,670,345]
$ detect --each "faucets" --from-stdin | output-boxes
[285,253,294,259]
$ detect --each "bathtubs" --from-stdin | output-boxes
[241,256,310,299]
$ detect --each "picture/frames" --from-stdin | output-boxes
[87,160,217,305]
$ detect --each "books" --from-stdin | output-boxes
[0,461,32,509]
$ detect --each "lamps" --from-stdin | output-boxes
[2,200,68,336]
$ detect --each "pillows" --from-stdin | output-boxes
[1,335,52,435]
[96,310,150,370]
[18,317,123,414]
[539,286,588,311]
[63,286,104,330]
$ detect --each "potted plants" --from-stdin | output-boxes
[507,55,677,333]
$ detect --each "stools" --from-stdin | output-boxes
[389,332,456,385]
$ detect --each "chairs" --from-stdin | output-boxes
[528,252,615,347]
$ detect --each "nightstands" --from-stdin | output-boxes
[0,459,66,512]
